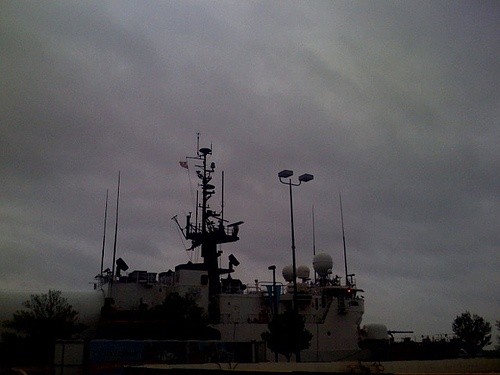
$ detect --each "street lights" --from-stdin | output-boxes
[278,169,314,362]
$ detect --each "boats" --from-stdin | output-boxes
[90,131,364,361]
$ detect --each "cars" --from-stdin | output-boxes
[0,364,39,375]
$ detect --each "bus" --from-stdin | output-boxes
[91,338,266,367]
